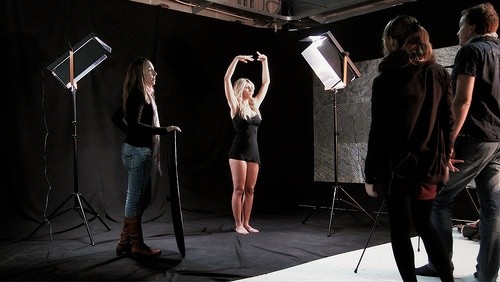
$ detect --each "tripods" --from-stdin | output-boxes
[303,89,380,237]
[26,87,110,246]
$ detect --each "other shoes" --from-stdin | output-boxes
[415,265,440,277]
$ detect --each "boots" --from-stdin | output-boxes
[116,215,127,256]
[126,216,161,257]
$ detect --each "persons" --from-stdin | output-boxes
[222,50,271,234]
[415,3,500,282]
[364,13,454,281]
[111,55,182,258]
